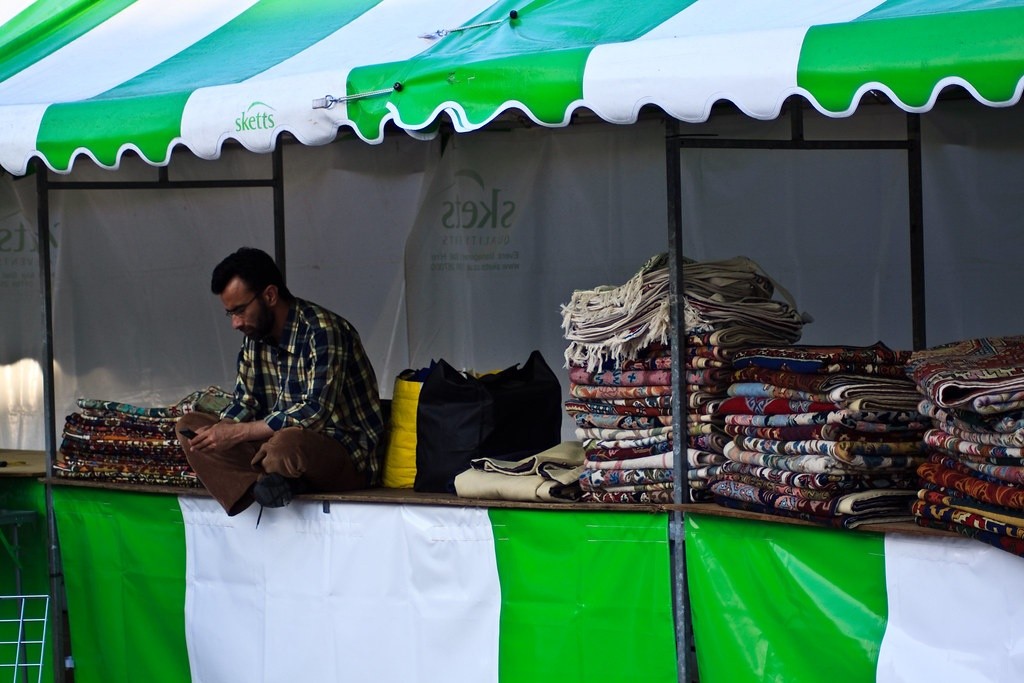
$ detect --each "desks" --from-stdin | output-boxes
[0,447,1024,683]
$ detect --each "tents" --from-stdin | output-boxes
[0,0,1024,683]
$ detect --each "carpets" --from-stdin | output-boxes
[52,386,232,487]
[454,251,1024,559]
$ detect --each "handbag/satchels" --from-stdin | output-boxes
[382,357,505,489]
[414,350,562,492]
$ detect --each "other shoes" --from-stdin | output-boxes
[253,473,294,508]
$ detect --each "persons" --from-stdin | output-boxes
[175,247,384,516]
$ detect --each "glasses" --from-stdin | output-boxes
[225,296,256,318]
